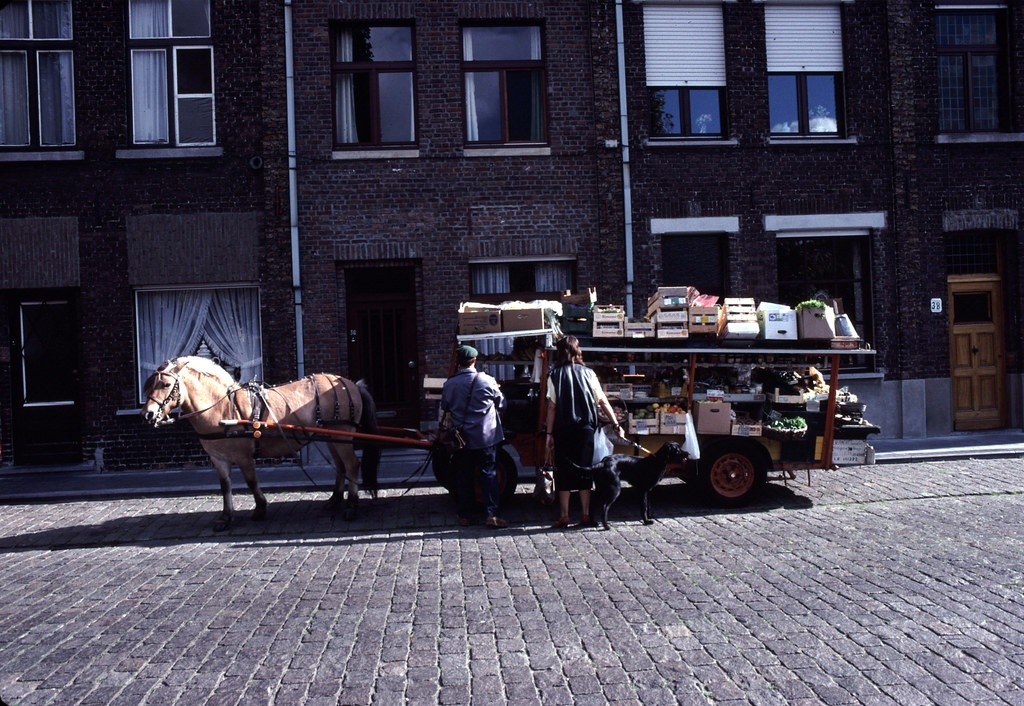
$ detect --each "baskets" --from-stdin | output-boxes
[597,395,629,425]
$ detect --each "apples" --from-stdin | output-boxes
[652,402,686,414]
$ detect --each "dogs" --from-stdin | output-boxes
[571,441,691,531]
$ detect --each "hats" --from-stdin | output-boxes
[459,345,478,357]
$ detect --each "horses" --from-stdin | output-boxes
[139,355,386,533]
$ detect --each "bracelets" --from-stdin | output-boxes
[546,433,552,435]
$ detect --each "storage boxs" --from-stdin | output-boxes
[458,311,502,334]
[693,401,731,435]
[647,286,719,338]
[659,425,686,435]
[590,305,626,323]
[502,309,545,331]
[661,413,686,424]
[759,308,797,339]
[721,297,761,338]
[622,323,656,338]
[799,307,836,339]
[833,440,867,465]
[731,424,763,436]
[593,323,624,337]
[598,381,829,403]
[627,413,659,434]
[561,309,593,335]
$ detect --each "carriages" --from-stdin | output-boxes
[139,287,883,534]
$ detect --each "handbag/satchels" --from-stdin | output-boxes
[592,426,614,466]
[533,446,557,507]
[435,424,466,460]
[681,413,700,460]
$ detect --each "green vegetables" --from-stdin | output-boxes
[795,300,828,318]
[766,416,807,430]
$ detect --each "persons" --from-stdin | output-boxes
[441,345,508,528]
[545,335,625,529]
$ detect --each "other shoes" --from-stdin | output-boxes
[456,514,470,526]
[580,516,598,527]
[486,516,506,528]
[554,517,569,529]
